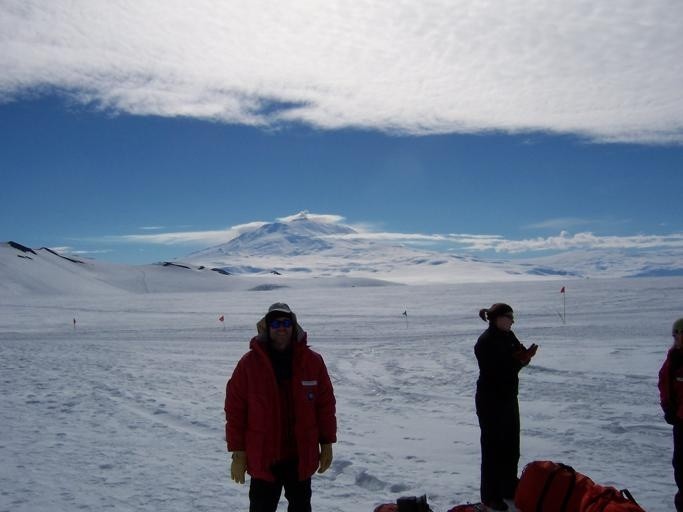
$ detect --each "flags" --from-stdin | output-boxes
[559,287,564,294]
[218,315,224,321]
[402,310,406,316]
[73,318,76,323]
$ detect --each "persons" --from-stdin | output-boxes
[222,300,339,512]
[657,317,682,512]
[471,299,540,512]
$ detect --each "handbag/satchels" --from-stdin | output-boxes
[515,460,594,512]
[580,485,644,512]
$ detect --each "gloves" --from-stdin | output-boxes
[512,344,538,366]
[230,451,248,484]
[317,443,332,473]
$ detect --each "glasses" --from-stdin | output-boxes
[501,314,515,320]
[269,317,292,330]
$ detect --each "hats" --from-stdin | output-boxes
[266,303,296,327]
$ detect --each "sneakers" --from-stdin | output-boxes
[486,495,508,511]
